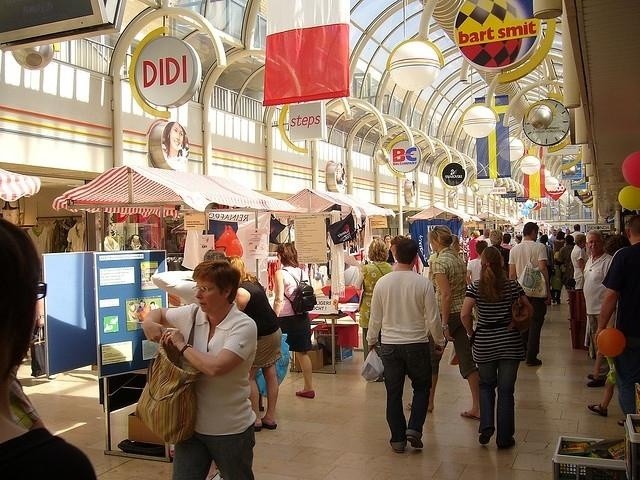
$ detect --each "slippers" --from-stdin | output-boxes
[253,418,276,432]
[461,412,480,421]
[526,358,542,366]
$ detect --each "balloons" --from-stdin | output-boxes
[618,151,640,210]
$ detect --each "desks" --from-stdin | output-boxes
[303,303,362,371]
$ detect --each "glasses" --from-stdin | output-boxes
[34,282,47,301]
[192,285,215,295]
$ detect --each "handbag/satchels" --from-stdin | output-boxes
[518,261,548,298]
[507,297,534,332]
[136,338,202,445]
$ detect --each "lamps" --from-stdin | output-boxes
[385,0,592,193]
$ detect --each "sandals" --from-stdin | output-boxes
[588,404,608,416]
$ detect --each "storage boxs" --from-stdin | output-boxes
[127,411,166,446]
[290,343,323,372]
[313,323,358,363]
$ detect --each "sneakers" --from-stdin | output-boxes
[587,375,604,387]
[390,435,423,453]
[496,438,515,449]
[479,427,495,444]
[296,389,315,398]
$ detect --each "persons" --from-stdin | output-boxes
[0,216,97,480]
[128,300,156,323]
[163,123,186,169]
[230,256,282,431]
[142,261,258,480]
[98,235,120,252]
[340,214,640,453]
[121,234,151,251]
[272,243,315,398]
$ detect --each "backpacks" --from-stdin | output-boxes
[282,268,317,313]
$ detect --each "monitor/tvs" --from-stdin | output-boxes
[0,0,120,46]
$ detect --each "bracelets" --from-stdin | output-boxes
[180,343,193,354]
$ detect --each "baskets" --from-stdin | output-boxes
[551,436,627,480]
